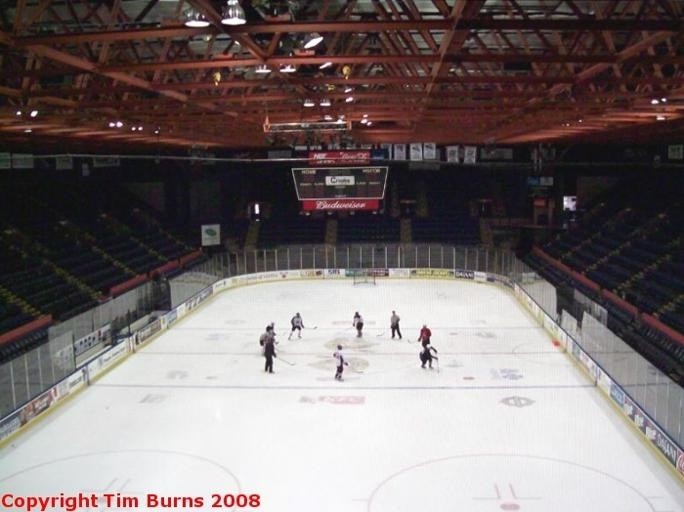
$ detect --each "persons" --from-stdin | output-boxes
[418,325,438,370]
[260,323,279,373]
[333,345,348,381]
[288,313,304,340]
[391,311,401,339]
[353,312,364,337]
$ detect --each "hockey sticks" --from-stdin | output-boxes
[276,356,297,366]
